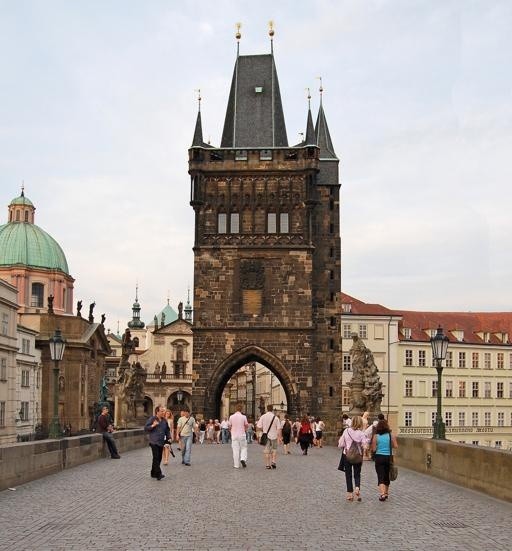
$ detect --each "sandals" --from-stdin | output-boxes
[346,490,389,502]
[266,463,276,469]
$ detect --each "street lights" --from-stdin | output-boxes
[429,323,449,439]
[45,327,67,438]
[177,388,183,405]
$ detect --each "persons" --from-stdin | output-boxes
[98,406,121,459]
[143,400,326,480]
[338,409,399,502]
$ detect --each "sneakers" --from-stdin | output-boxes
[163,458,191,466]
[241,460,247,467]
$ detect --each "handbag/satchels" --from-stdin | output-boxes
[259,433,267,445]
[345,441,363,465]
[390,462,398,481]
